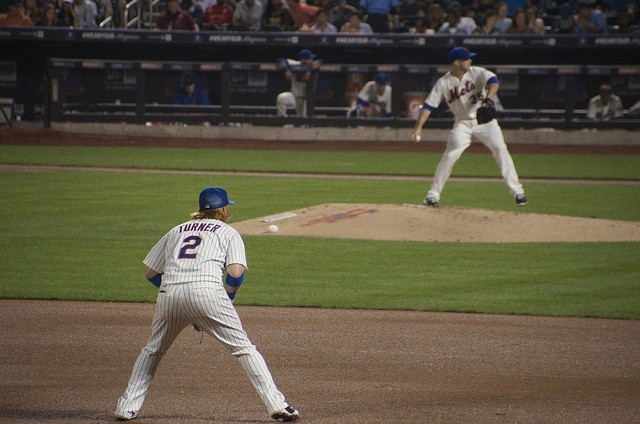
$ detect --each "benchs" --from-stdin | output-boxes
[65,98,640,130]
[1,0,640,63]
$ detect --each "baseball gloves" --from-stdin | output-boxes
[477,98,496,124]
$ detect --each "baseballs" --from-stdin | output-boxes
[269,225,277,233]
[412,134,420,142]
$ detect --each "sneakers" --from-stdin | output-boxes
[271,404,300,421]
[516,193,527,206]
[422,197,439,206]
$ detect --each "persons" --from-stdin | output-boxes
[172,75,211,105]
[114,188,301,420]
[347,72,394,116]
[410,46,528,207]
[588,83,623,122]
[275,49,324,116]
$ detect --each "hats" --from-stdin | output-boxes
[376,72,388,84]
[447,46,477,62]
[199,186,235,210]
[298,48,316,58]
